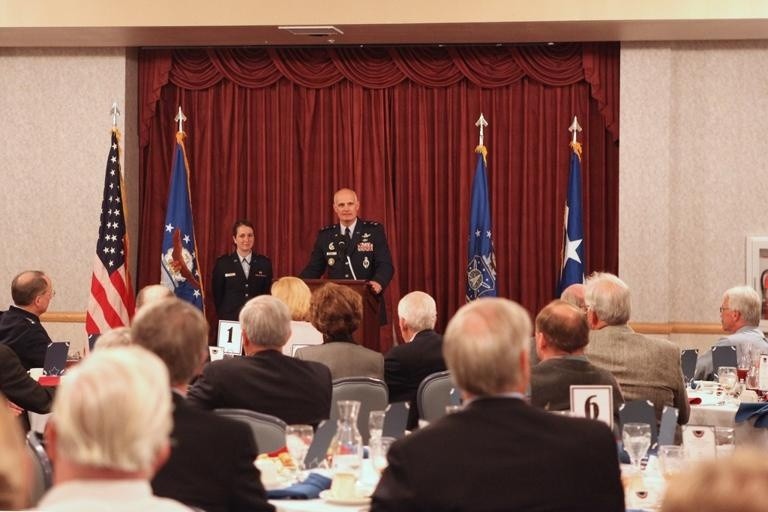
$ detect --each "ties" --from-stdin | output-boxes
[344,227,352,254]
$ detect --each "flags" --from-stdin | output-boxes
[160,132,205,318]
[557,141,585,298]
[465,145,498,302]
[85,126,136,350]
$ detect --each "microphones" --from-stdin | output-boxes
[332,234,352,259]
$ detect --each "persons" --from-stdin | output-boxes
[298,188,394,327]
[1,270,768,511]
[211,219,273,321]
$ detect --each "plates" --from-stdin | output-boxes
[318,489,373,505]
[687,393,720,405]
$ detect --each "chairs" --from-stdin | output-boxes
[416,370,460,423]
[24,431,52,490]
[329,376,390,444]
[214,409,291,455]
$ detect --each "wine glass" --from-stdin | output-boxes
[370,437,396,479]
[285,424,314,479]
[623,424,652,475]
[714,427,736,459]
[659,445,682,479]
[368,410,386,439]
[333,440,362,474]
[746,366,758,390]
[718,366,738,400]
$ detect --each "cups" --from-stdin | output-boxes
[330,472,359,500]
[254,460,273,489]
[27,368,44,382]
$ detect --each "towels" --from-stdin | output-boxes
[267,473,329,501]
[734,402,768,429]
